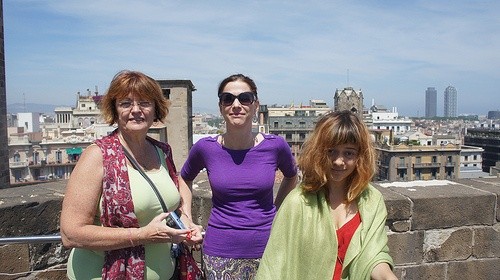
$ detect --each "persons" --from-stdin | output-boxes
[59,69,203,280]
[254,109,398,280]
[177,73,300,280]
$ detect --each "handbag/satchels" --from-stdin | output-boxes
[174,242,202,280]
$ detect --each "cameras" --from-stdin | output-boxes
[166,212,185,230]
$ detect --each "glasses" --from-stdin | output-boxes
[219,92,256,106]
[118,101,153,109]
